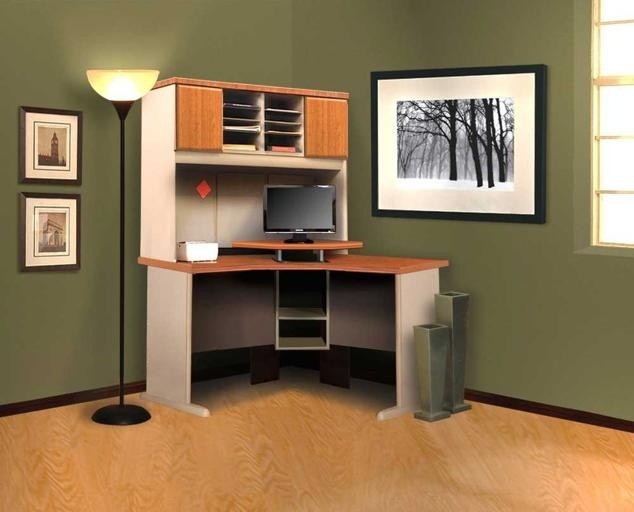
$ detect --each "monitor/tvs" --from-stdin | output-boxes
[262,184,336,244]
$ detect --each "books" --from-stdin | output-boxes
[223,123,261,134]
[223,102,260,109]
[266,145,298,153]
[223,143,257,151]
